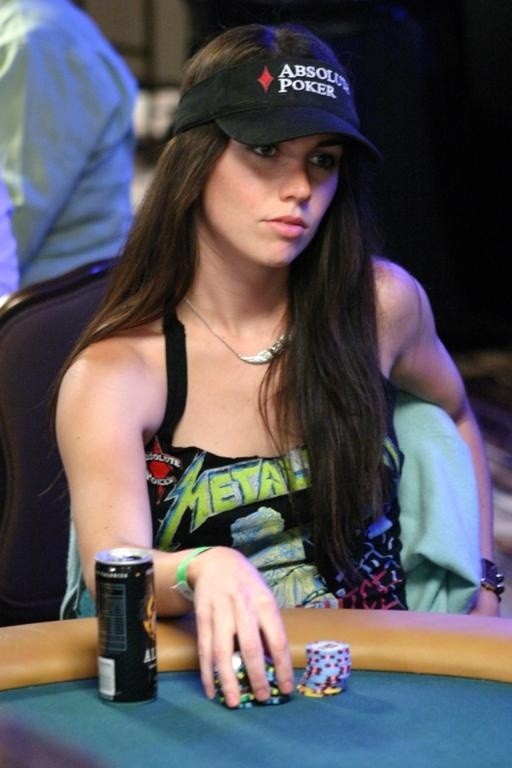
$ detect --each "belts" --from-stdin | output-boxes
[0,253,115,318]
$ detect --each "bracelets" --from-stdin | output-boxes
[171,545,211,603]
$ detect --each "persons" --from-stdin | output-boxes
[41,20,506,710]
[0,0,141,626]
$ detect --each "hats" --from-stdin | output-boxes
[172,61,383,161]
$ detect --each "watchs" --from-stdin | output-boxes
[478,553,504,603]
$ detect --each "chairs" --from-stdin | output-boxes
[0,245,143,638]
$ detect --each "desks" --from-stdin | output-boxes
[1,605,512,768]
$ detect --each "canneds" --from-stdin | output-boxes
[95,547,157,706]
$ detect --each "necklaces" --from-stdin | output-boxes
[181,282,297,365]
